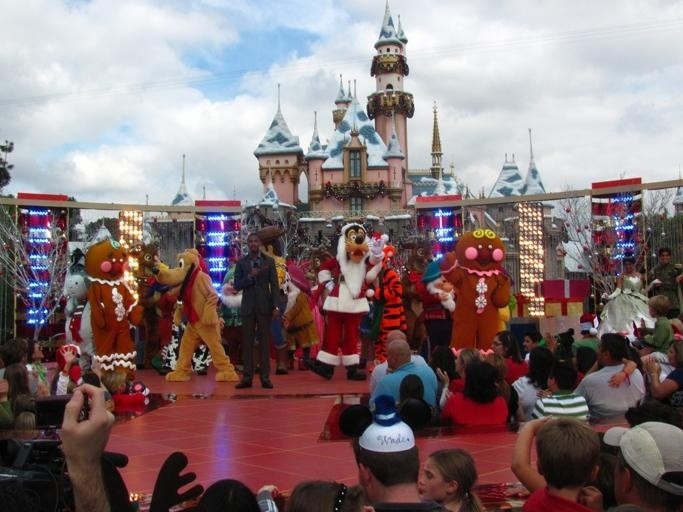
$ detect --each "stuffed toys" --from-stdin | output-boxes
[284,225,514,382]
[62,224,515,388]
[62,225,239,382]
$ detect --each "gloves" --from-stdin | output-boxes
[640,278,662,295]
[601,288,621,301]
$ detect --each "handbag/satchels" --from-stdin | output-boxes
[0,439,73,512]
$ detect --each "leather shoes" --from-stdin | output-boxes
[262,381,273,388]
[234,383,251,388]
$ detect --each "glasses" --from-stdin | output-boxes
[494,342,502,346]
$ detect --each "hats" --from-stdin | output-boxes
[339,395,432,452]
[556,329,574,348]
[579,312,600,336]
[257,484,287,512]
[55,347,77,371]
[602,421,682,496]
[1,339,29,367]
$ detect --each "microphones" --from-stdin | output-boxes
[254,263,258,280]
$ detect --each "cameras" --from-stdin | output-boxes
[33,393,88,429]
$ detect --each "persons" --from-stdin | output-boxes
[1,321,683,512]
[233,233,282,389]
[641,294,673,353]
[648,248,683,320]
[597,253,661,341]
